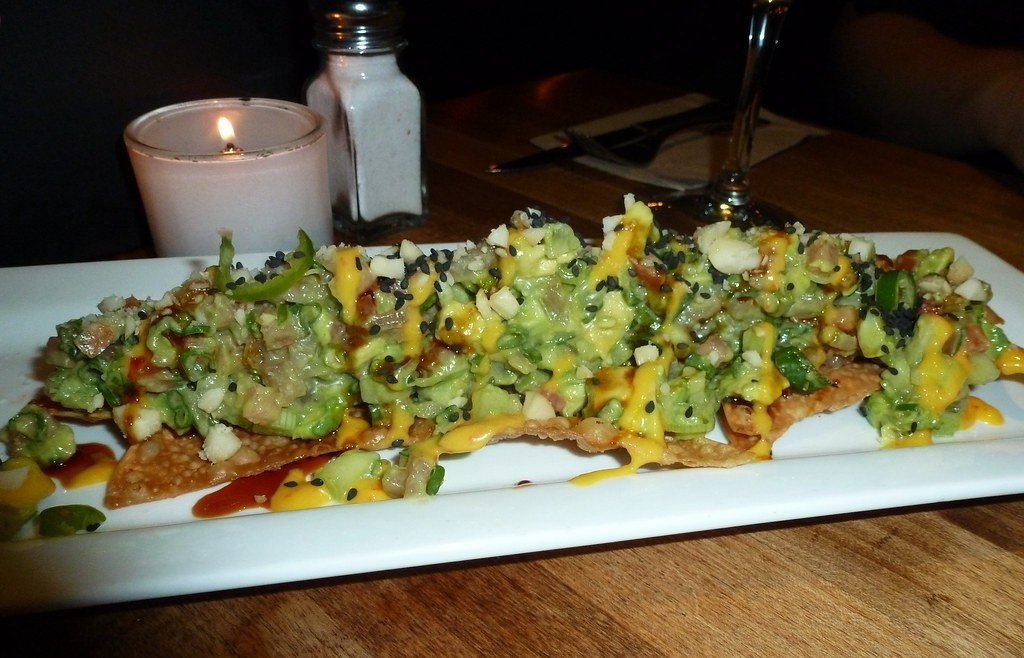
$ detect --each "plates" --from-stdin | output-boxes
[0,232,1024,606]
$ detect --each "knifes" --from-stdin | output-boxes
[488,92,751,172]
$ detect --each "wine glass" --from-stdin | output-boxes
[627,0,813,233]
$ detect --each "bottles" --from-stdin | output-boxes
[306,1,421,238]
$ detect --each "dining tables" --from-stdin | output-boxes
[0,74,1024,658]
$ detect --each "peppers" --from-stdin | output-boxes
[35,227,917,538]
